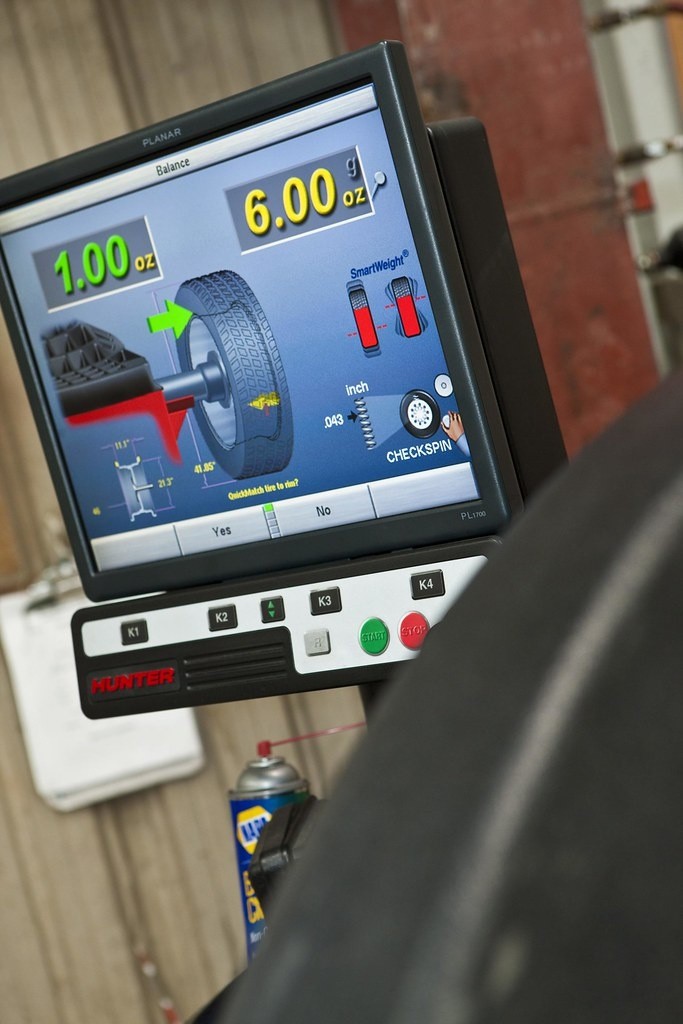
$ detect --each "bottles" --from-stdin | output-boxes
[228,741,310,967]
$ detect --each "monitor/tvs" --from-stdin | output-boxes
[0,38,523,603]
